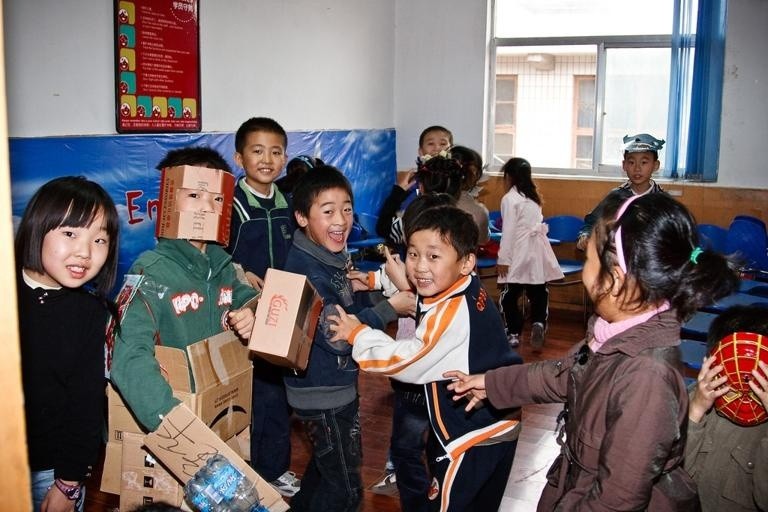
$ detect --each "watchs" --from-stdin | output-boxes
[54,478,83,501]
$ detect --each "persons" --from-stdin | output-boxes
[281,166,418,512]
[368,193,455,497]
[398,126,454,207]
[443,197,740,511]
[224,117,294,292]
[14,175,116,512]
[444,146,491,246]
[104,148,260,434]
[493,157,566,354]
[375,155,462,264]
[576,134,669,252]
[680,302,767,512]
[329,206,521,511]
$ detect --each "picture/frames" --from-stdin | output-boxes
[113,1,202,134]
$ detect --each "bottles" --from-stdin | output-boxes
[182,469,233,512]
[205,453,270,512]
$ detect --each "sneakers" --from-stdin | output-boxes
[530,322,545,348]
[268,471,301,497]
[371,473,399,496]
[507,333,519,347]
